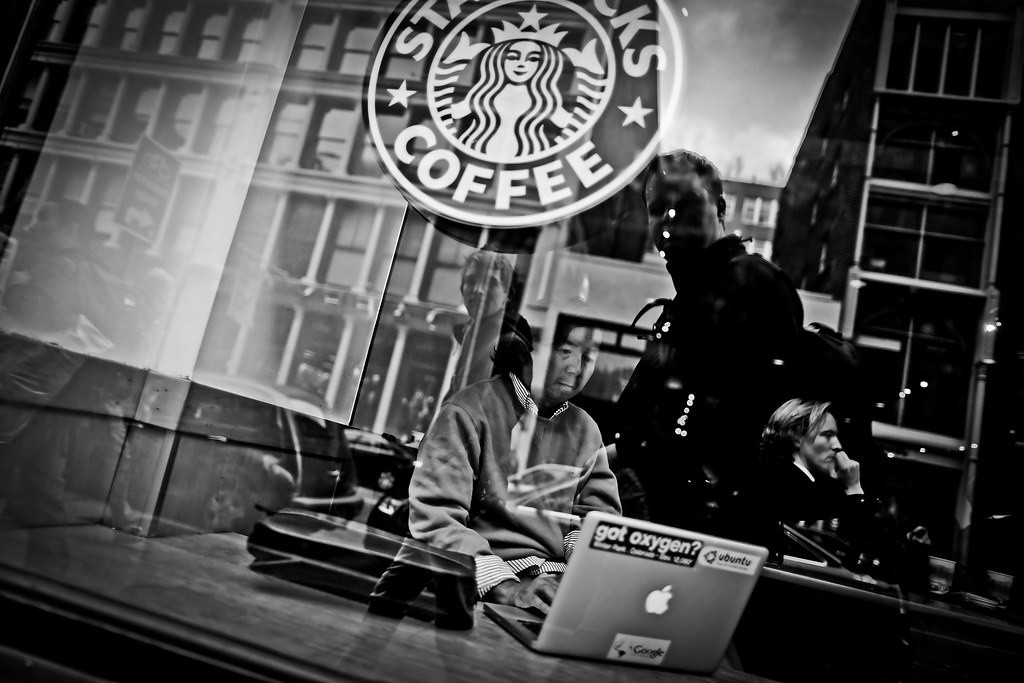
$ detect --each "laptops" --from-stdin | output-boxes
[481,509,771,673]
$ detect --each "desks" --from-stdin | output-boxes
[0,526,772,683]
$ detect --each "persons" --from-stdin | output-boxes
[338,249,534,683]
[612,146,804,539]
[872,472,954,598]
[408,294,622,614]
[714,398,911,683]
[1,197,147,535]
